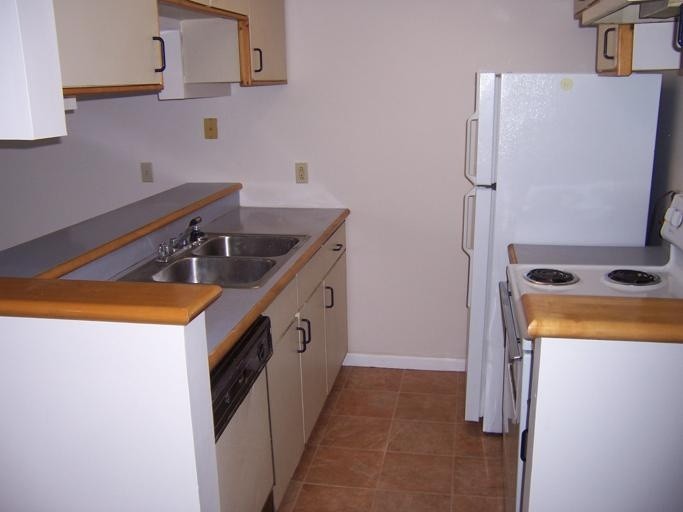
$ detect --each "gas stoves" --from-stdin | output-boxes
[510,262,683,291]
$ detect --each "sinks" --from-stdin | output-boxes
[185,232,310,257]
[124,256,281,289]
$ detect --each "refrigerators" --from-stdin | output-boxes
[462,72,663,438]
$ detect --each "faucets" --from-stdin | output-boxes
[165,235,207,260]
[174,216,202,246]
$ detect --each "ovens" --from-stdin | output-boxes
[498,280,532,512]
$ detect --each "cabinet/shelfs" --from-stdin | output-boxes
[54,0,288,96]
[573,0,683,76]
[263,221,349,512]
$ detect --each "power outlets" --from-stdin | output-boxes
[295,162,308,183]
